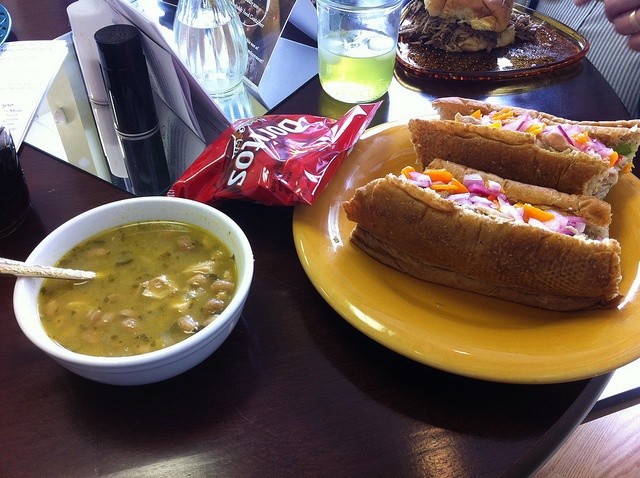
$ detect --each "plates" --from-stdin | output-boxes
[398,0,589,82]
[292,119,640,385]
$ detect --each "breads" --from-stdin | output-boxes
[342,159,624,310]
[424,0,516,52]
[407,96,640,205]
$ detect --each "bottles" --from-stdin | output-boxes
[171,0,253,123]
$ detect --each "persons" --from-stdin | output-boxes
[517,1,640,110]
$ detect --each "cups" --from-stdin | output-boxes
[314,0,403,104]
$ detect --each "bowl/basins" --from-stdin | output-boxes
[13,196,254,387]
